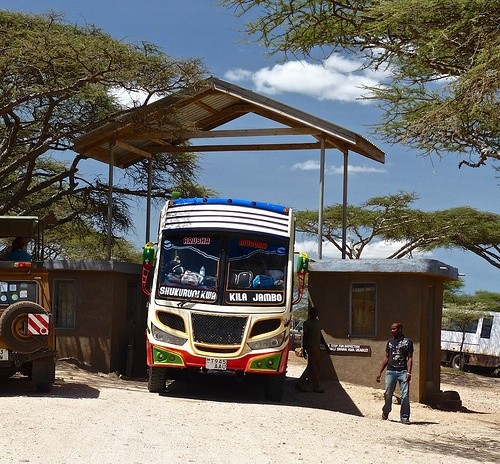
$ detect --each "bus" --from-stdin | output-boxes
[146,197,297,401]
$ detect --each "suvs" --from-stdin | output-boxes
[0,216,56,391]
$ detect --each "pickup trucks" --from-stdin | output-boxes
[441,309,500,371]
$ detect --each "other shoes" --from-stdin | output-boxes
[382,413,388,420]
[401,418,410,424]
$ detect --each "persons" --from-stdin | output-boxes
[294,307,331,393]
[0,236,31,261]
[376,323,415,424]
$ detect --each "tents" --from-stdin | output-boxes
[68,77,387,259]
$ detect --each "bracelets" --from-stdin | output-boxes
[406,372,412,376]
[325,347,329,350]
